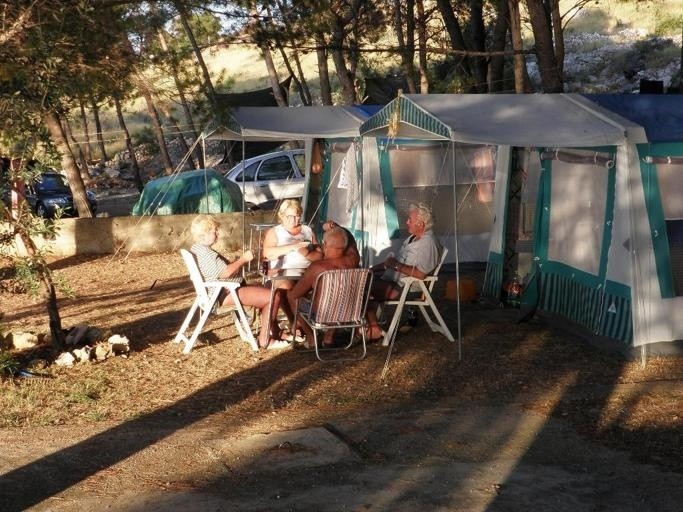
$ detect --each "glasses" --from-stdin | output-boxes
[284,214,300,220]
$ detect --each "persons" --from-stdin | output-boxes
[191,199,442,351]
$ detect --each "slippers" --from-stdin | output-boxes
[266,324,360,351]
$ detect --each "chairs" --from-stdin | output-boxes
[178,244,453,364]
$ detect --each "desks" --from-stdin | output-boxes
[246,223,314,273]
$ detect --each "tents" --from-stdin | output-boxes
[132,169,249,215]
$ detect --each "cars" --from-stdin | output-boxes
[225,148,306,211]
[24,171,97,219]
